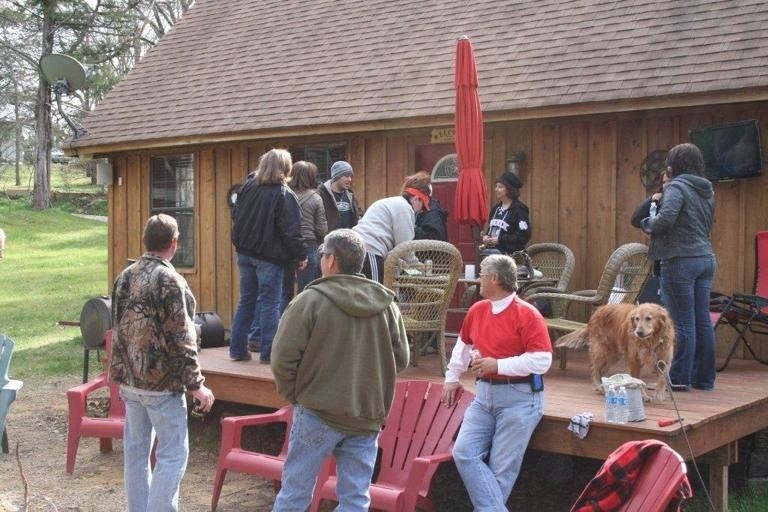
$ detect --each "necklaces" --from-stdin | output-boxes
[333,190,346,203]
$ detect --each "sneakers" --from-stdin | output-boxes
[228,353,252,361]
[647,382,690,391]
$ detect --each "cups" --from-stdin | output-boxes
[469,350,482,361]
[465,263,476,278]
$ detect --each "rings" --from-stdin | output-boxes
[478,368,482,373]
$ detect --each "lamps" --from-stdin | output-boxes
[503,149,525,181]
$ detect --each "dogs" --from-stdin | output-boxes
[553,302,676,403]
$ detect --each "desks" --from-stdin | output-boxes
[392,271,558,367]
[81,346,105,383]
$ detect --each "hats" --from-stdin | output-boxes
[330,160,354,183]
[501,171,524,188]
[404,187,431,212]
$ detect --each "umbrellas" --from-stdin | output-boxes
[454,35,492,302]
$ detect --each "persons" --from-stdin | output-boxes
[648,143,717,392]
[112,213,215,512]
[279,160,326,314]
[317,159,364,231]
[244,155,263,353]
[629,171,674,304]
[351,172,431,284]
[478,171,529,256]
[229,148,309,365]
[269,226,410,511]
[440,255,553,512]
[410,186,448,354]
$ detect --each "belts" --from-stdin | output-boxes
[476,375,532,386]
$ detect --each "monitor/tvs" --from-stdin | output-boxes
[688,119,764,180]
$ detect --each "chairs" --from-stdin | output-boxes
[0,333,24,457]
[306,377,477,512]
[64,324,158,478]
[209,398,294,511]
[706,230,767,377]
[520,241,656,372]
[587,436,689,512]
[456,239,575,353]
[381,239,465,380]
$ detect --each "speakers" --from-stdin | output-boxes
[96,164,114,185]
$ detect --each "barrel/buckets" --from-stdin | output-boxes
[623,374,646,422]
[196,310,226,348]
[78,294,113,346]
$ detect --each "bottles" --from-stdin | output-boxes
[425,259,433,277]
[604,384,630,425]
[649,201,658,218]
